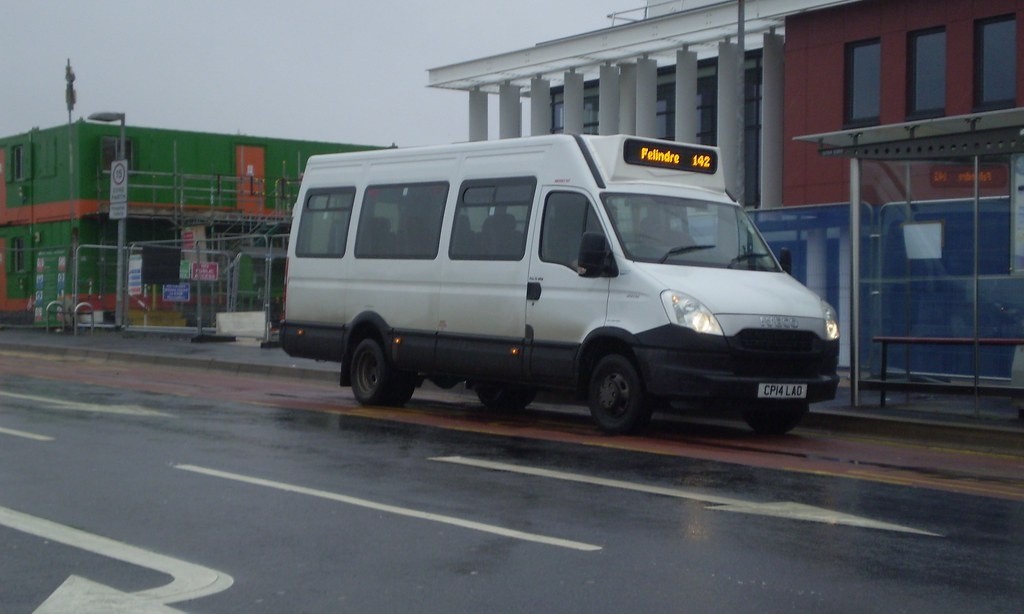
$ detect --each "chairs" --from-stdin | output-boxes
[329,210,522,262]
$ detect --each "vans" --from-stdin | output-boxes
[278,134,842,438]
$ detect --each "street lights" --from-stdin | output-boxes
[89,111,126,330]
